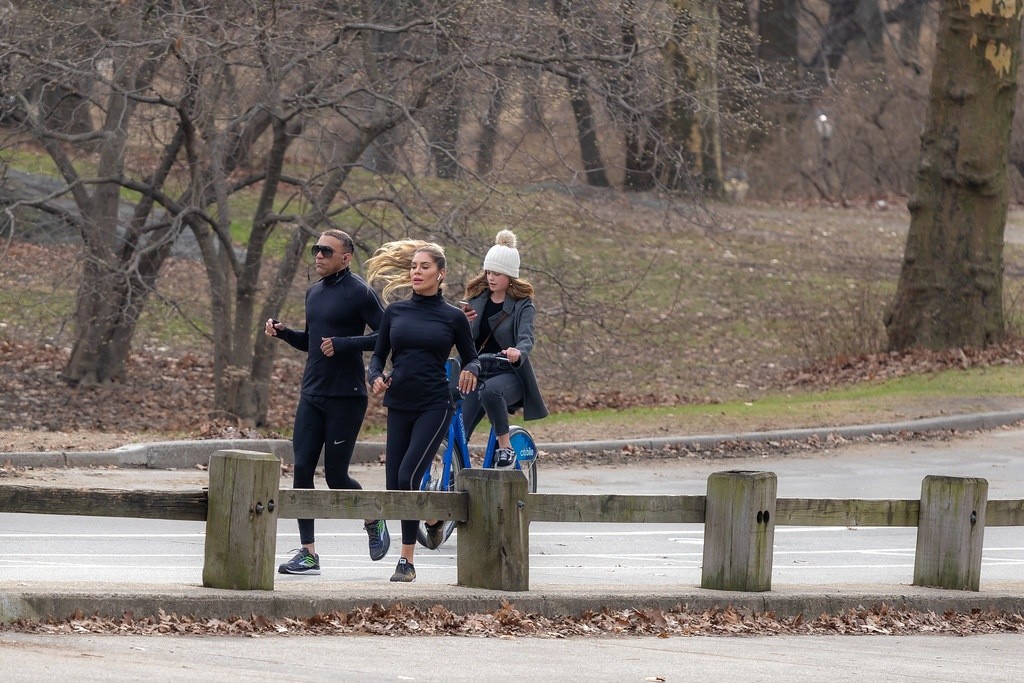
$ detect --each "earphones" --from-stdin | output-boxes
[437,274,441,281]
[343,258,346,260]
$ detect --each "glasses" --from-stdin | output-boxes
[310,245,347,258]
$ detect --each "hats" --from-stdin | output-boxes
[484,229,521,278]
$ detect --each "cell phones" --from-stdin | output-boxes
[458,301,474,313]
[383,368,395,383]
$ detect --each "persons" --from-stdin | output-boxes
[444,229,550,492]
[261,227,385,577]
[363,237,484,583]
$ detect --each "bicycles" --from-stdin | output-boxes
[410,351,540,549]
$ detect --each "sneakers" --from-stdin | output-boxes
[390,557,416,582]
[494,447,517,471]
[424,520,450,550]
[278,547,321,575]
[363,519,390,561]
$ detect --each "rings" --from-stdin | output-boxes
[464,378,470,382]
